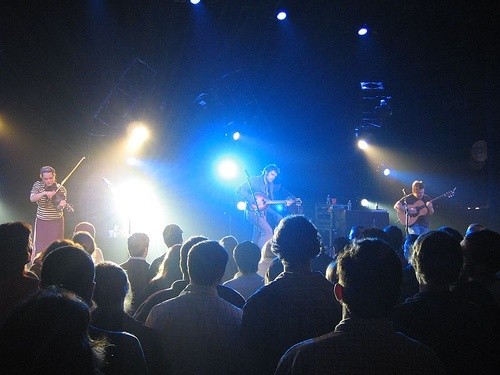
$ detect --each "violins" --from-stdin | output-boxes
[43,183,74,215]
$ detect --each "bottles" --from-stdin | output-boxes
[327,194,330,205]
[375,202,378,210]
[348,200,352,210]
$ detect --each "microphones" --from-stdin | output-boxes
[402,189,406,195]
[244,169,249,178]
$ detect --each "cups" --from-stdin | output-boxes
[331,198,337,205]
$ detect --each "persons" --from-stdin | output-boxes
[30,165,68,255]
[273,239,442,375]
[242,214,343,375]
[0,222,498,375]
[144,240,243,375]
[235,164,295,249]
[393,180,434,235]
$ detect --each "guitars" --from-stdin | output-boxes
[250,192,303,211]
[397,187,458,226]
